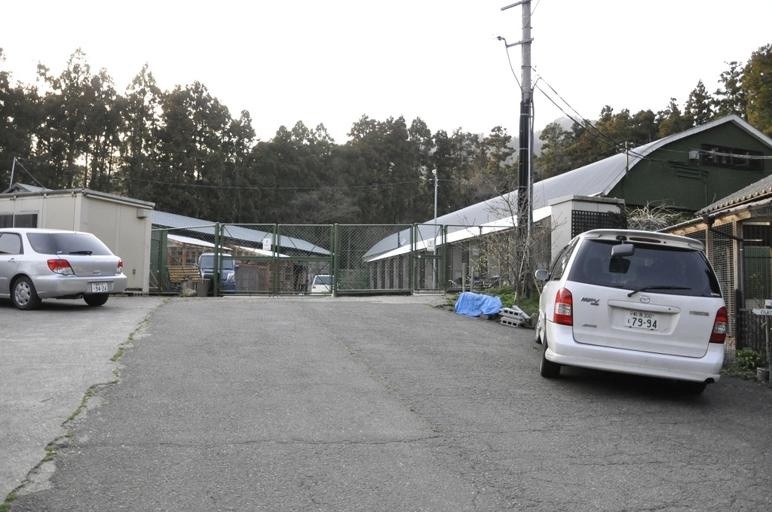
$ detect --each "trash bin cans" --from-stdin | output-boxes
[192,278,211,296]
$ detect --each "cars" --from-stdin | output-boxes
[533,227,729,396]
[0,227,129,310]
[193,251,240,294]
[310,274,341,297]
